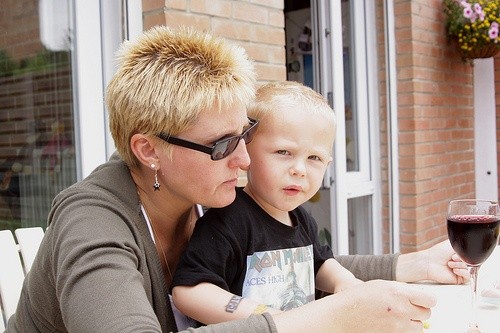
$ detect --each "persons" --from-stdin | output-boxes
[0,24,472,333]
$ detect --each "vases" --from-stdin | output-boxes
[452,41,498,59]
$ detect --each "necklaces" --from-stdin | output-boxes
[130,191,196,284]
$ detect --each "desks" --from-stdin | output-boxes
[408,237,500,333]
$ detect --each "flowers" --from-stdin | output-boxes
[442,0,500,67]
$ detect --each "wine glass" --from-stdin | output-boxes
[447,199,500,333]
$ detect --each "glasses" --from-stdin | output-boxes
[155,115,260,161]
